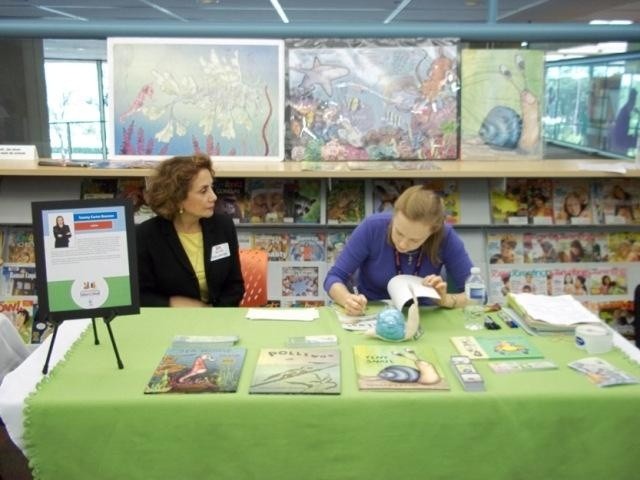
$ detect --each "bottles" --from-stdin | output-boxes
[462,267,486,331]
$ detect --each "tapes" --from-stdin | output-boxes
[575,325,613,355]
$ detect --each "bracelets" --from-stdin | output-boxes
[449,294,458,308]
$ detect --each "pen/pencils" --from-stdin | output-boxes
[485,311,518,329]
[353,286,365,316]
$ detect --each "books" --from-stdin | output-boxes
[450,332,546,361]
[497,304,640,341]
[137,338,247,394]
[484,178,640,303]
[79,175,161,227]
[38,156,154,170]
[0,223,60,346]
[247,345,343,397]
[333,274,441,332]
[213,176,366,305]
[372,179,460,225]
[351,342,452,393]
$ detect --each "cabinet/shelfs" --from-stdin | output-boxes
[0,159,639,345]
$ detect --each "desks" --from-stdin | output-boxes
[0,302,640,479]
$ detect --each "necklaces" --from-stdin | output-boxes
[405,252,421,266]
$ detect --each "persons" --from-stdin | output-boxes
[322,183,483,317]
[134,154,247,311]
[608,88,640,161]
[52,215,72,248]
